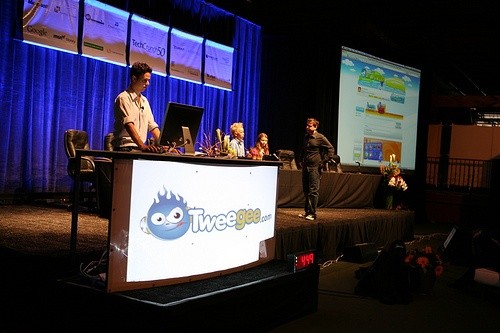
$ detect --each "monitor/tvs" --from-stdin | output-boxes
[158,102,204,147]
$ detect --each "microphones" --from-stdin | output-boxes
[355,161,362,174]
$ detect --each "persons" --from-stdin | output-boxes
[227,122,270,160]
[111,61,161,152]
[297,118,334,220]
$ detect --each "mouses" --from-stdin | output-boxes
[147,148,162,153]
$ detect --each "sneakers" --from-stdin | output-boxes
[298,211,316,221]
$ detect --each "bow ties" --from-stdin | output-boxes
[235,138,243,145]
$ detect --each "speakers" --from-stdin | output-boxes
[372,240,414,304]
[343,241,377,264]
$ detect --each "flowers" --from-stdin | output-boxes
[379,153,408,210]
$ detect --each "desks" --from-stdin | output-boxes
[70,149,283,292]
[278,169,384,208]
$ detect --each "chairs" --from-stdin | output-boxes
[276,149,298,170]
[64,129,114,214]
